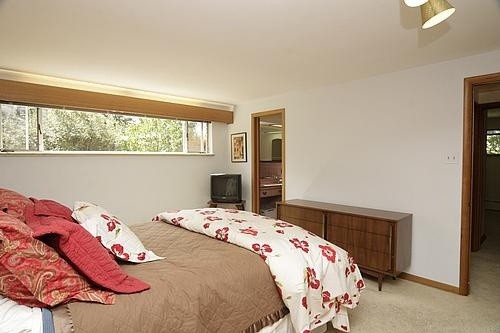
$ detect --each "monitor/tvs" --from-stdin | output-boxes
[210,173,242,204]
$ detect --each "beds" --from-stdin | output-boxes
[0,207,366,333]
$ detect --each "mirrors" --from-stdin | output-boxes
[260,114,282,162]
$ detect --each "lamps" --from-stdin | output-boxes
[404,0,455,29]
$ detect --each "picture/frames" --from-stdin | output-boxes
[231,132,247,163]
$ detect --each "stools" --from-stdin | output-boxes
[207,200,246,211]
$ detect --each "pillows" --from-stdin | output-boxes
[0,188,166,309]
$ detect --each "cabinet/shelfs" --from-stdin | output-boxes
[260,178,282,197]
[276,199,413,291]
